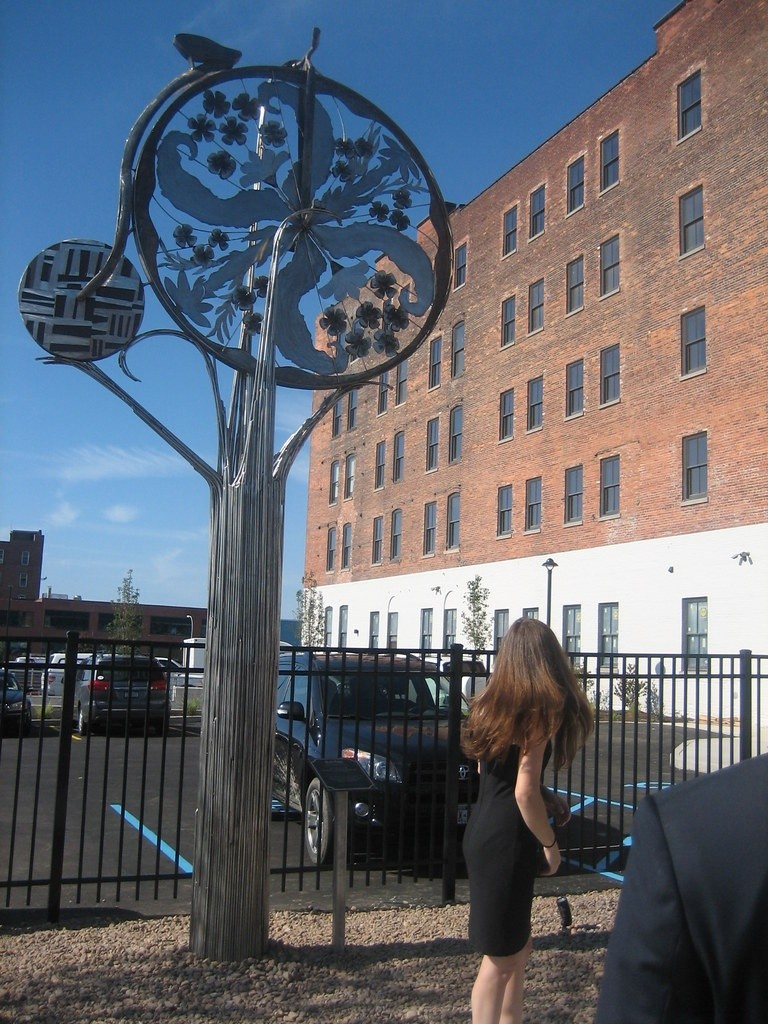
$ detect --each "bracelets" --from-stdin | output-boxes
[542,836,558,848]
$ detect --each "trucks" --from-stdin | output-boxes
[182,638,294,666]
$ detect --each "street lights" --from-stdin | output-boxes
[187,615,193,640]
[543,557,557,629]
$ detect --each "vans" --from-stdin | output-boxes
[16,657,45,663]
[41,653,101,696]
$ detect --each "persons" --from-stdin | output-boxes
[461,618,594,1024]
[596,752,768,1024]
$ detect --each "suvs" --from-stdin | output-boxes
[60,654,170,735]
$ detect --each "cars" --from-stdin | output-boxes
[0,669,32,734]
[273,650,482,873]
[156,657,204,688]
